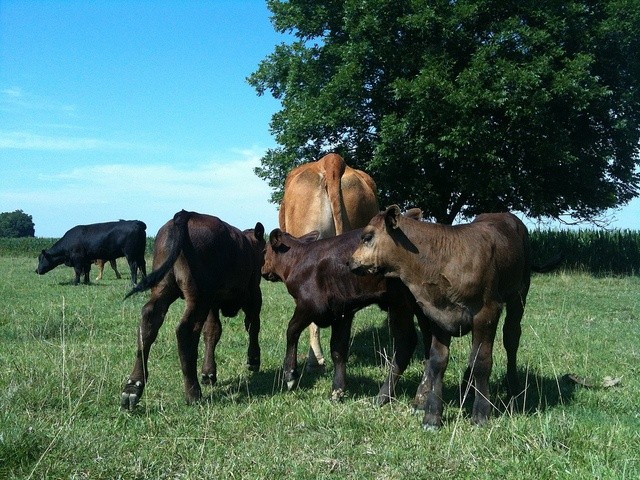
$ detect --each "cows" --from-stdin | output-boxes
[351,205,531,431]
[120,209,267,412]
[260,230,433,410]
[279,153,381,374]
[64,258,122,280]
[35,219,146,286]
